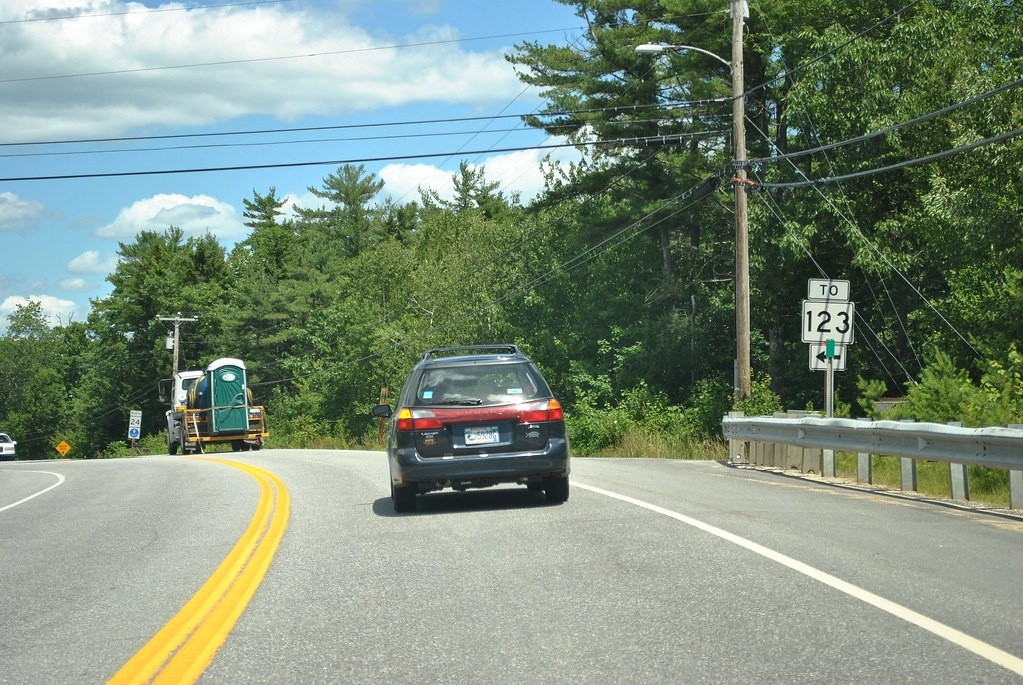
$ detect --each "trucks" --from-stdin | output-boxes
[159,371,270,455]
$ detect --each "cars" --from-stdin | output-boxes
[0,433,18,461]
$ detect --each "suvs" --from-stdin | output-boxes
[371,345,570,513]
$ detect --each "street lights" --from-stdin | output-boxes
[635,43,751,408]
